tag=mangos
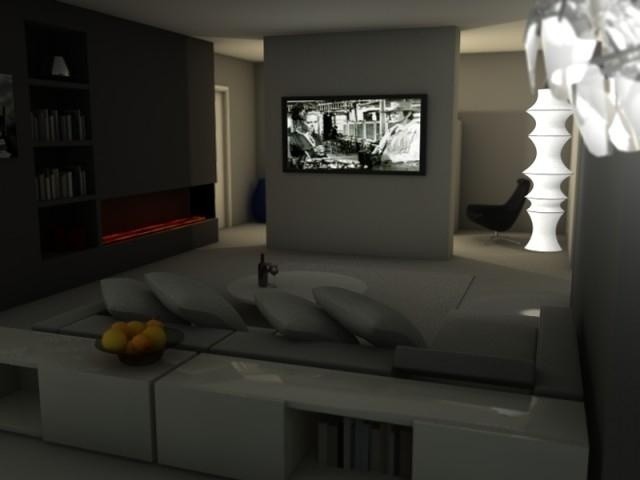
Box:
[102,319,166,353]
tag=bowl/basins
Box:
[95,325,186,366]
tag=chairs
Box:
[465,178,530,246]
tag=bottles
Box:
[257,253,268,287]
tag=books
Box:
[29,105,97,203]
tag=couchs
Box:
[31,296,582,400]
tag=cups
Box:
[303,100,386,169]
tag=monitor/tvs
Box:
[280,93,429,177]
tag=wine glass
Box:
[269,264,279,288]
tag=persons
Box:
[370,99,420,164]
[288,109,328,170]
